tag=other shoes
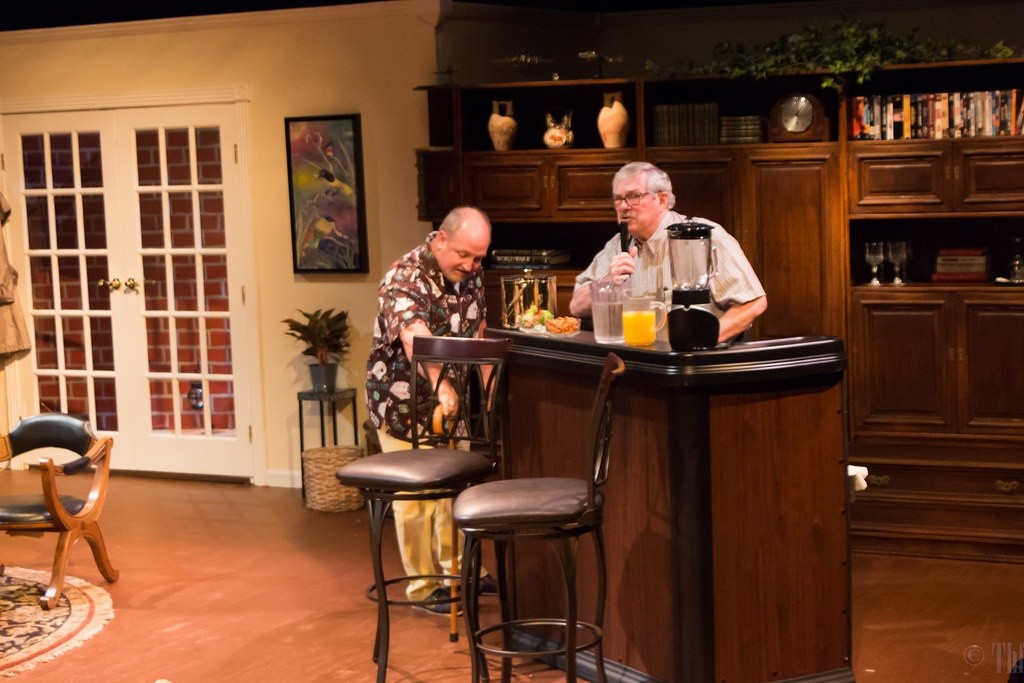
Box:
[411,587,465,616]
[443,575,500,595]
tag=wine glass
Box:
[866,243,883,287]
[888,242,906,287]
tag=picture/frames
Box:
[283,112,370,274]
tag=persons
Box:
[366,206,497,618]
[569,162,768,343]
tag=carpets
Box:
[0,565,116,679]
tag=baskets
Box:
[302,446,368,512]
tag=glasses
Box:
[608,190,664,208]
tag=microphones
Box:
[620,221,629,254]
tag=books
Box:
[647,103,762,146]
[851,89,1024,139]
[491,250,569,269]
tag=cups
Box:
[590,280,624,345]
[623,296,668,346]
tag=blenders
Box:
[665,222,728,352]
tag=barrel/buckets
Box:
[500,269,557,329]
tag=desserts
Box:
[544,315,580,335]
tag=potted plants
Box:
[281,307,353,394]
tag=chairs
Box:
[334,335,512,683]
[452,351,628,683]
[0,412,120,611]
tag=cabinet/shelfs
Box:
[296,387,358,499]
[415,56,1024,566]
